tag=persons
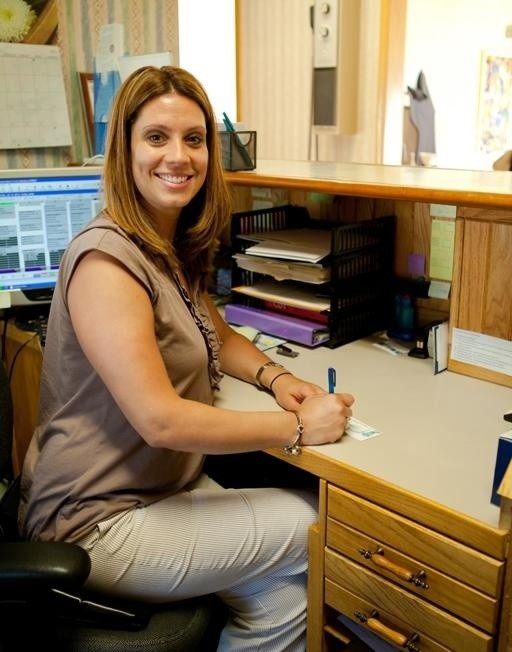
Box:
[14,65,358,651]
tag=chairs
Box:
[0,362,221,651]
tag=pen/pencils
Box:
[328,368,336,394]
[223,112,253,167]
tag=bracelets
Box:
[267,372,293,392]
[253,361,285,392]
[280,406,305,457]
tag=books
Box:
[222,223,379,348]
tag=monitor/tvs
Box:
[0,166,107,301]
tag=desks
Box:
[0,151,511,651]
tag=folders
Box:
[225,304,330,347]
